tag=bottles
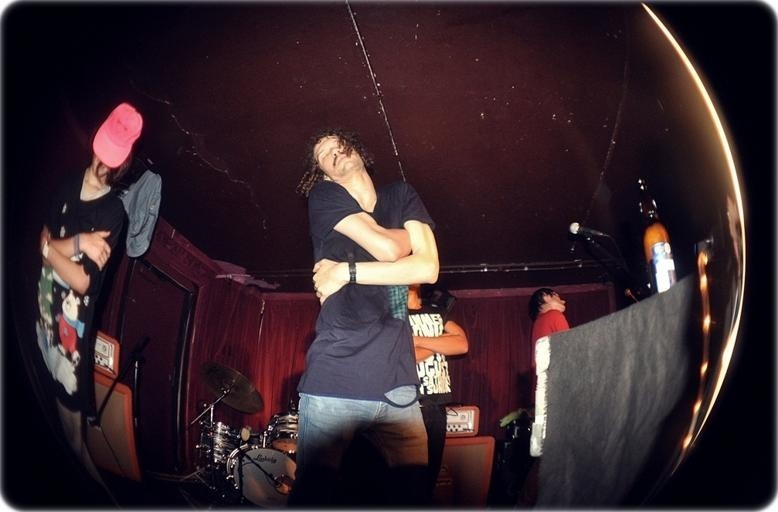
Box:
[651,242,677,295]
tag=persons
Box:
[521,285,576,465]
[40,99,146,470]
[282,129,448,493]
[400,279,470,465]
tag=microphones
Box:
[118,335,150,381]
[570,220,609,239]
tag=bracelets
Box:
[347,262,358,285]
[42,240,50,259]
[72,233,81,256]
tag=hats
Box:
[92,102,144,170]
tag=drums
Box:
[269,410,297,454]
[226,443,295,509]
[199,418,263,477]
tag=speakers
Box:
[434,436,496,508]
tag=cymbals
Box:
[200,361,263,414]
